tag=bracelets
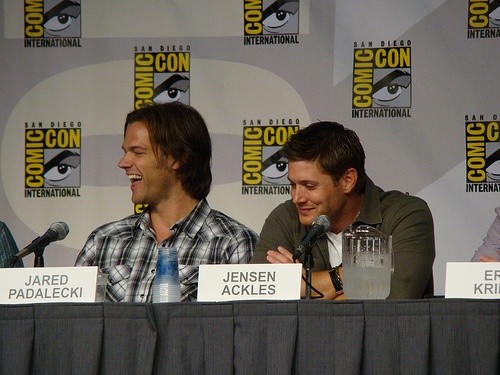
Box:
[329,267,344,296]
[335,267,343,289]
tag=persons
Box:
[249,121,436,300]
[470,207,500,262]
[72,100,260,303]
[0,220,27,268]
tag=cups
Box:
[152,247,181,303]
[96,274,106,304]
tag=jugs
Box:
[342,225,395,300]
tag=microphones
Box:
[292,215,331,260]
[10,222,70,266]
